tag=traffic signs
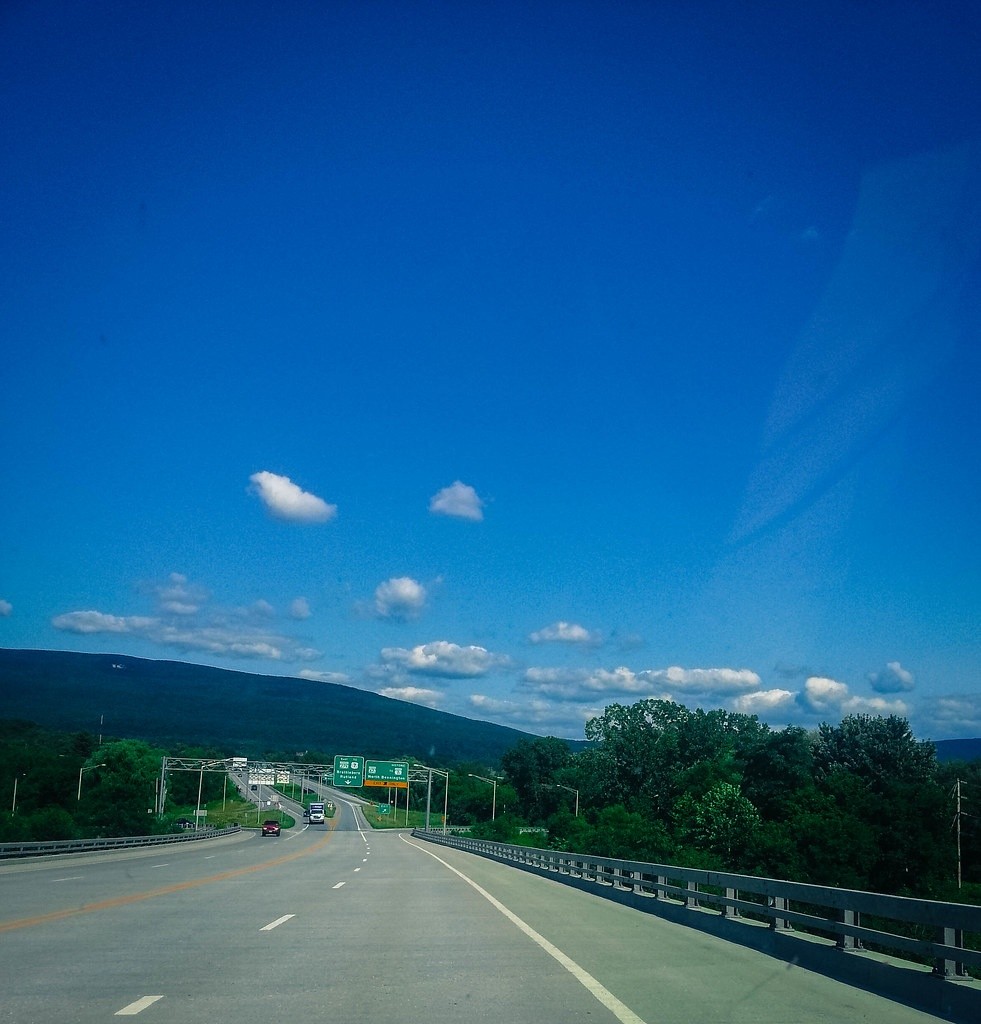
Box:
[364,759,409,788]
[333,756,364,787]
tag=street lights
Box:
[557,784,578,816]
[155,774,174,812]
[468,774,496,821]
[12,772,26,811]
[195,758,230,831]
[78,764,106,801]
[414,764,449,835]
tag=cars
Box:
[238,774,242,777]
[251,785,258,791]
[175,818,195,828]
[261,821,282,837]
[304,811,310,817]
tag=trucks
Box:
[308,802,325,824]
